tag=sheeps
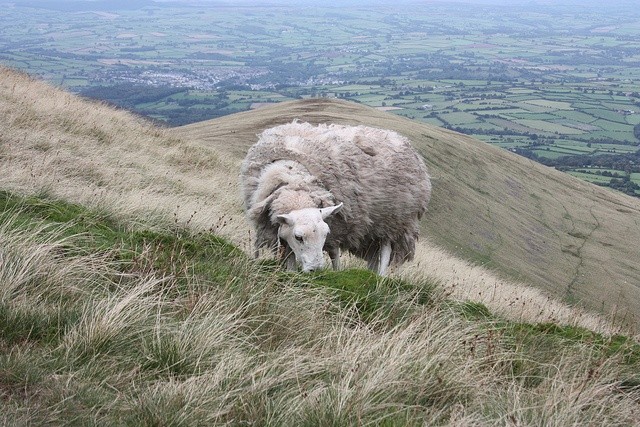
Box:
[240,119,431,280]
